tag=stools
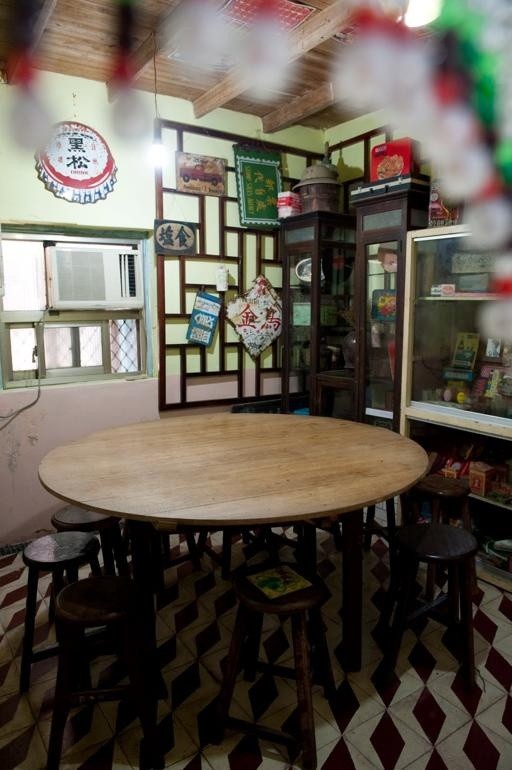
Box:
[410,475,481,612]
[377,521,480,705]
[44,576,165,770]
[203,556,338,770]
[120,516,366,580]
[43,502,132,629]
[16,529,103,710]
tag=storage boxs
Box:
[292,300,338,328]
[449,252,501,274]
[349,172,431,206]
[456,273,492,293]
[369,136,423,181]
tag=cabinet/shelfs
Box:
[277,190,429,434]
[400,226,512,594]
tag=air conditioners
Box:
[42,241,146,312]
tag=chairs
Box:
[229,398,283,414]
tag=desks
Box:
[34,407,433,742]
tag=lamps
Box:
[139,29,174,171]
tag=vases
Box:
[341,330,377,371]
